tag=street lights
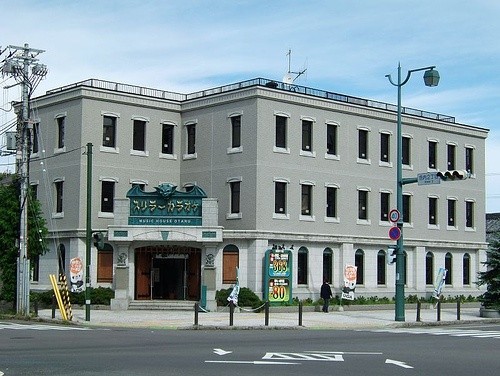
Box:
[384,61,440,321]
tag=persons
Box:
[320,279,333,313]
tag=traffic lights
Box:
[436,169,469,181]
